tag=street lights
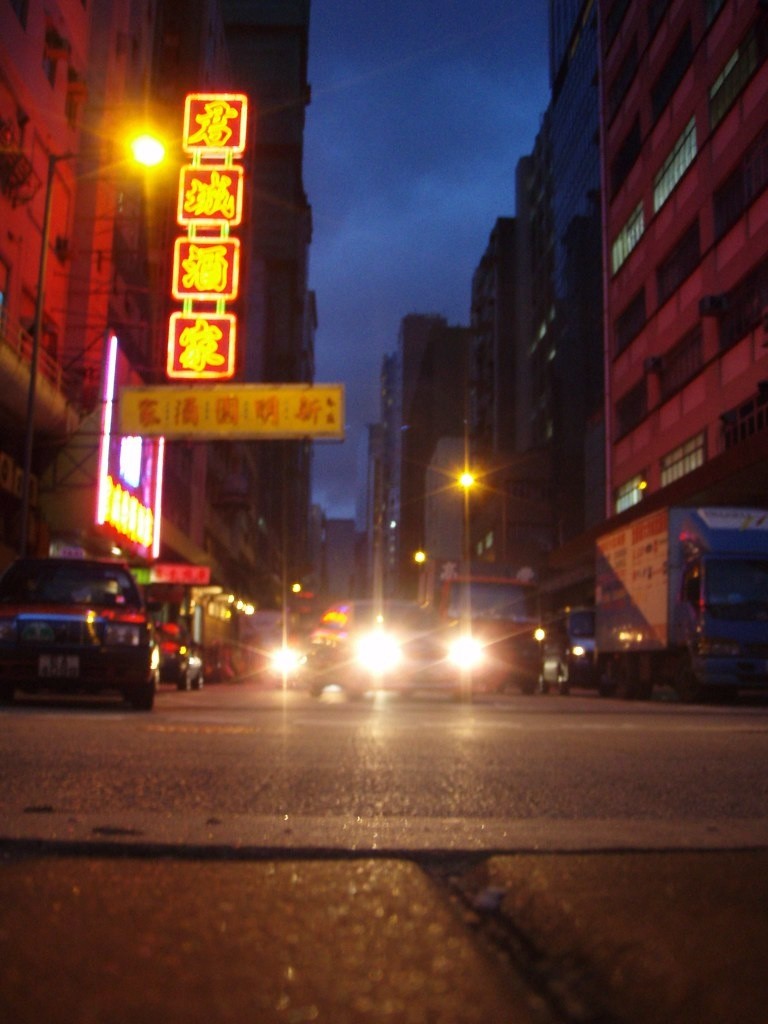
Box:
[19,133,168,560]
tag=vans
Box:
[538,606,595,695]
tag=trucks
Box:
[596,504,768,700]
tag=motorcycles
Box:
[441,577,543,696]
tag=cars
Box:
[0,557,158,709]
[310,596,474,705]
[153,629,205,690]
